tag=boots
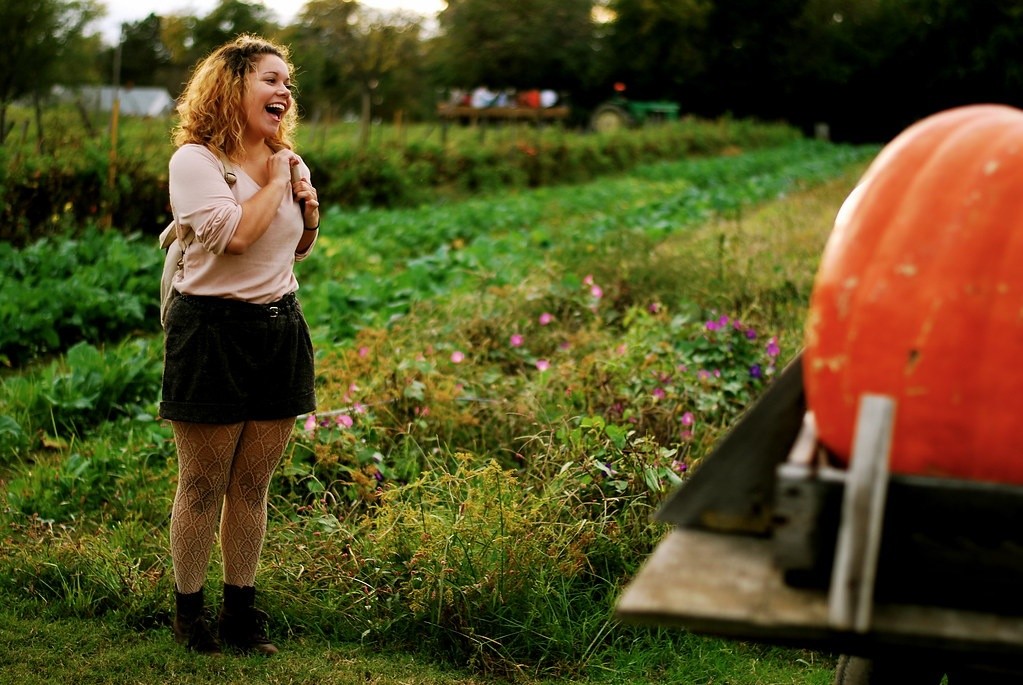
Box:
[173,581,221,655]
[217,584,271,648]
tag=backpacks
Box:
[159,145,302,330]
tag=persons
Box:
[159,36,320,655]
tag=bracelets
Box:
[304,222,319,231]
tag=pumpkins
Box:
[806,103,1022,488]
